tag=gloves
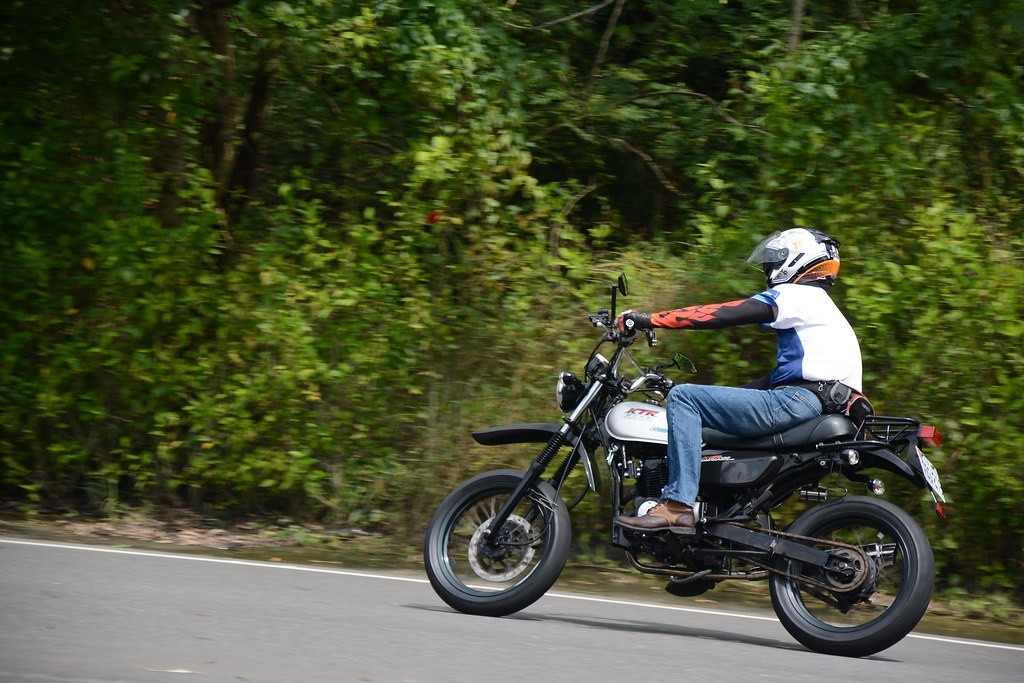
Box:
[616,309,653,336]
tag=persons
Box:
[613,227,864,598]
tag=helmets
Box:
[746,227,841,288]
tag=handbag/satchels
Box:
[793,379,876,424]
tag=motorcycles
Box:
[423,272,949,657]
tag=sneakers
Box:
[664,572,730,597]
[615,499,696,535]
